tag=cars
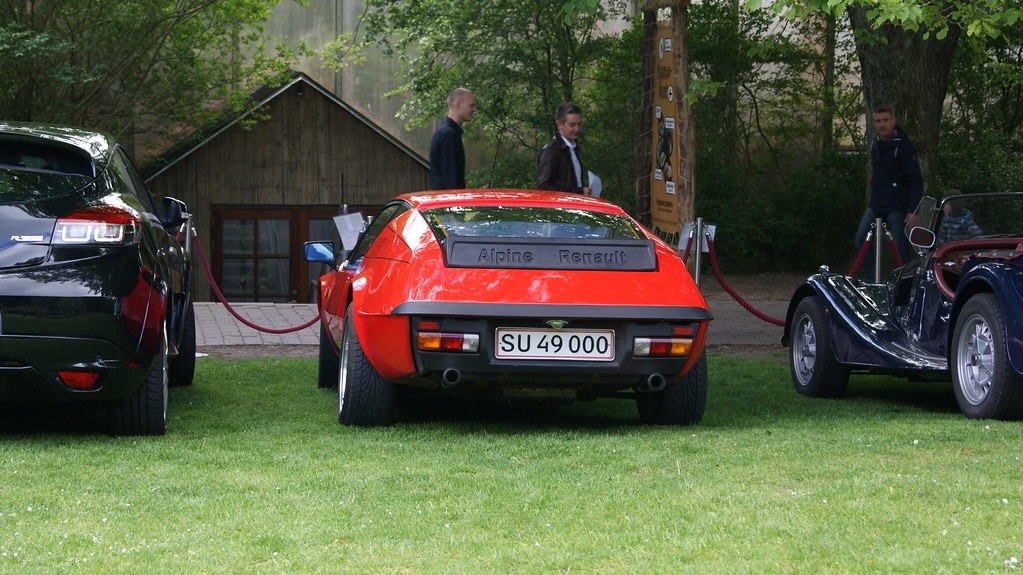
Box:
[1,122,198,434]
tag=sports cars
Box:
[780,190,1023,422]
[300,187,715,427]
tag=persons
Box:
[536,103,592,196]
[937,189,982,249]
[430,88,476,191]
[854,103,922,267]
